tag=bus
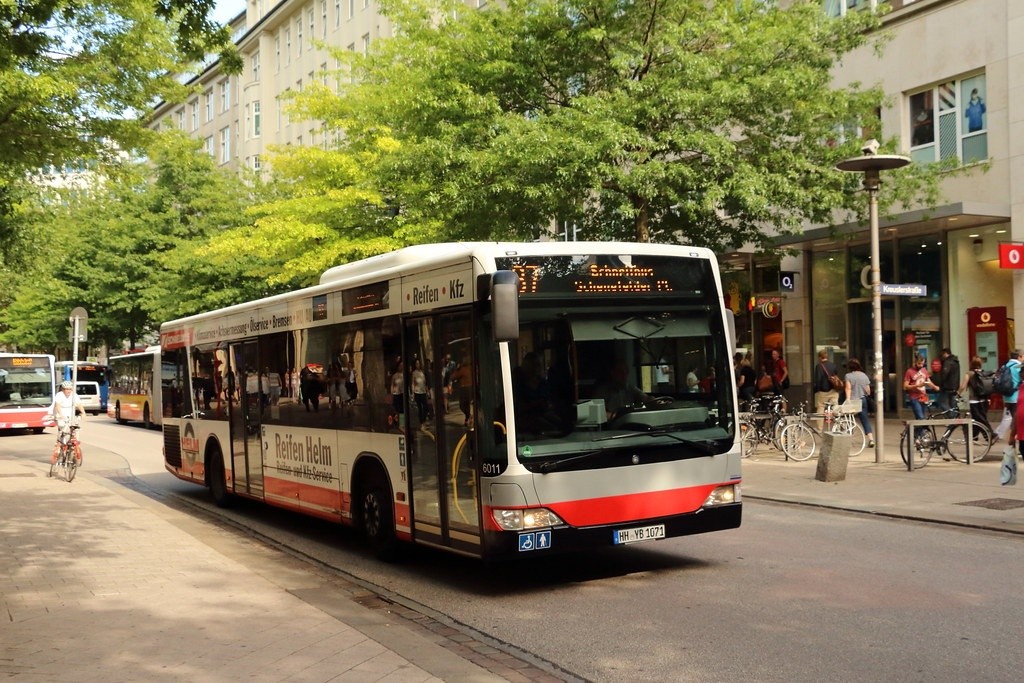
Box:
[103,344,164,430]
[0,353,56,434]
[158,238,745,559]
[54,360,109,411]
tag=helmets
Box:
[63,381,73,389]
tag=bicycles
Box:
[52,415,83,482]
[739,393,867,463]
[898,389,993,470]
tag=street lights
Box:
[835,139,913,464]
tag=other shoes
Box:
[990,434,998,444]
[868,441,874,448]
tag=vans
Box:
[56,380,102,416]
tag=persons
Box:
[815,350,840,433]
[934,348,960,430]
[366,352,476,435]
[514,352,570,428]
[49,381,86,478]
[844,358,875,447]
[900,354,940,439]
[995,348,1024,461]
[170,378,185,417]
[965,88,986,133]
[956,357,998,445]
[734,349,789,414]
[655,351,717,393]
[190,360,358,436]
[603,359,655,420]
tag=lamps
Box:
[920,239,927,247]
[973,238,983,254]
[936,238,943,246]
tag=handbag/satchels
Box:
[999,446,1017,485]
[781,375,790,390]
[829,376,843,388]
[757,374,773,390]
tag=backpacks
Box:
[968,368,993,396]
[991,363,1022,397]
